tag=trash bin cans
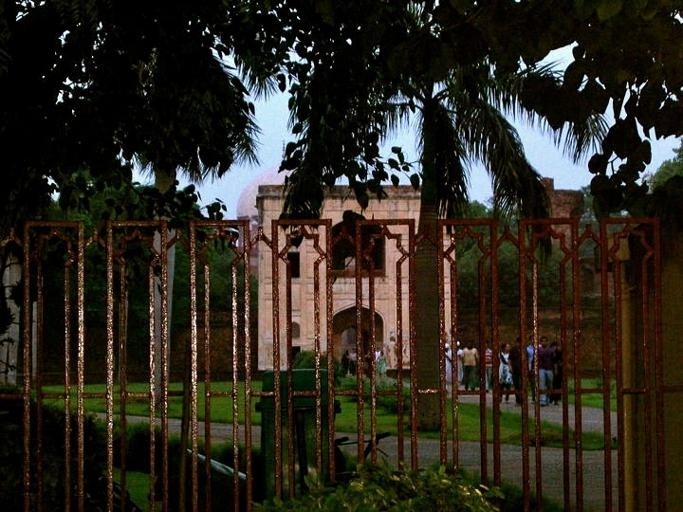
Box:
[256,368,341,501]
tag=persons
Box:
[445,334,563,407]
[342,336,398,377]
